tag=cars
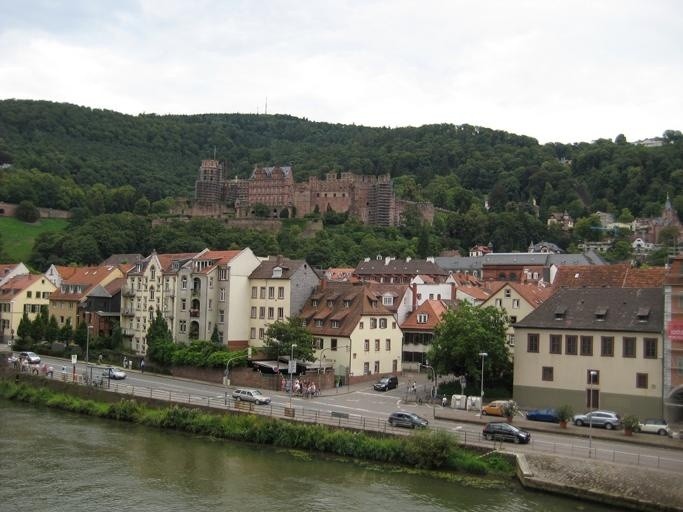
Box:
[104,368,125,379]
[19,351,40,364]
[482,400,510,416]
[526,408,560,423]
[632,418,668,435]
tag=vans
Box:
[374,375,398,392]
[232,388,271,405]
[387,412,428,429]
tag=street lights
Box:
[589,371,598,458]
[85,325,94,382]
[290,343,298,408]
[478,352,488,399]
[422,363,436,403]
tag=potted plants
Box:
[555,403,577,429]
[618,415,642,436]
[503,406,513,422]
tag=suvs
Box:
[572,409,620,429]
[483,422,530,443]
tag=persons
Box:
[122,355,128,369]
[98,352,104,365]
[280,377,321,400]
[140,357,145,374]
[9,357,66,378]
[440,391,447,409]
[414,380,417,388]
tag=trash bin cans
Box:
[223,377,230,385]
[71,355,77,364]
